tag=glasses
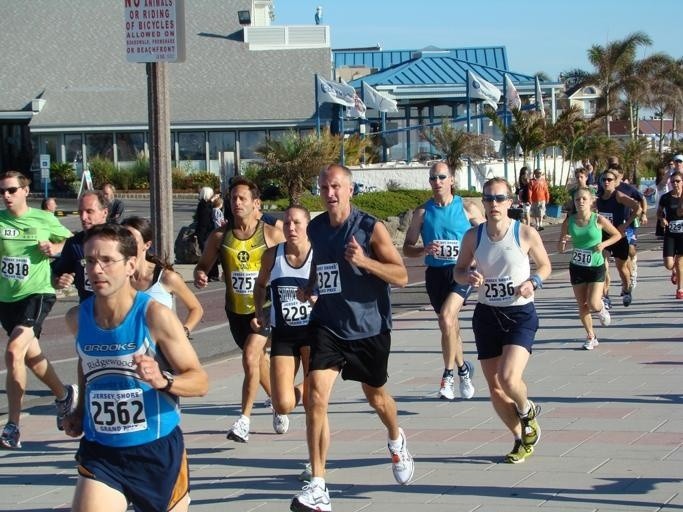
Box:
[429,175,452,181]
[602,178,613,182]
[80,256,130,268]
[671,180,682,183]
[0,185,26,195]
[483,195,510,203]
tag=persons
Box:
[516,153,683,351]
[404,163,488,402]
[64,222,209,510]
[194,181,291,443]
[291,163,416,511]
[195,186,284,281]
[452,177,553,464]
[1,168,79,451]
[41,177,205,341]
[252,203,314,480]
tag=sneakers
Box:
[0,422,22,449]
[298,462,313,481]
[270,398,289,434]
[461,362,475,400]
[388,427,414,487]
[518,400,540,447]
[504,441,533,463]
[291,483,332,512]
[54,384,79,430]
[583,255,683,351]
[228,420,248,444]
[438,376,455,401]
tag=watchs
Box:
[158,370,174,392]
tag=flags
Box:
[337,76,367,119]
[468,70,502,109]
[361,80,399,112]
[315,76,354,106]
[536,75,547,125]
[505,73,523,117]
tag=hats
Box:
[608,156,619,165]
[673,155,683,162]
[198,186,214,203]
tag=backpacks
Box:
[174,227,201,264]
